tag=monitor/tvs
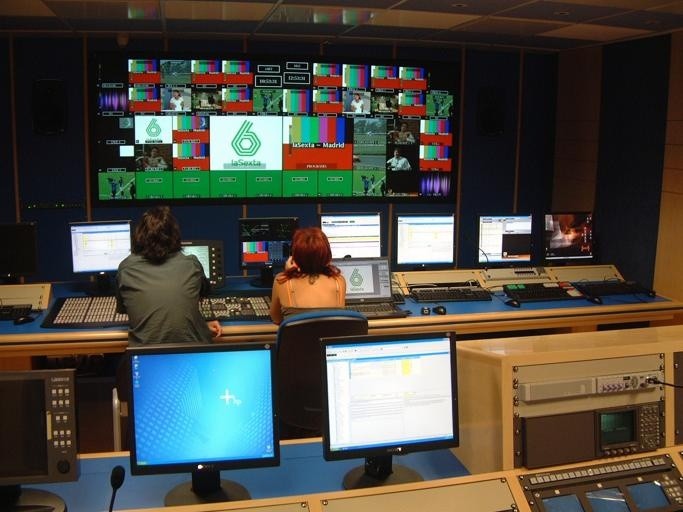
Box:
[393,211,455,271]
[473,211,536,270]
[91,49,460,209]
[0,368,78,512]
[180,239,226,289]
[318,328,461,490]
[319,212,384,258]
[66,220,134,294]
[0,221,37,276]
[237,216,300,287]
[540,210,596,265]
[124,342,282,507]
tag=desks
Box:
[0,264,683,370]
[1,429,682,512]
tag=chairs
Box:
[270,309,369,442]
[111,388,130,452]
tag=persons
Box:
[143,147,168,171]
[263,91,283,112]
[199,95,216,107]
[110,177,135,200]
[270,227,346,321]
[351,93,365,113]
[432,95,452,116]
[170,90,185,110]
[388,122,416,145]
[387,148,411,171]
[114,206,223,344]
[362,174,386,196]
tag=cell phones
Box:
[422,306,431,315]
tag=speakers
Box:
[31,78,69,134]
[475,86,503,140]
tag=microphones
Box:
[108,462,127,512]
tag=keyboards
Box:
[503,280,586,301]
[570,281,645,297]
[412,289,493,302]
[0,304,32,320]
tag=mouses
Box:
[591,296,602,304]
[504,299,520,308]
[649,290,655,298]
[14,315,35,325]
[433,305,446,314]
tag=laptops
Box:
[327,257,405,319]
